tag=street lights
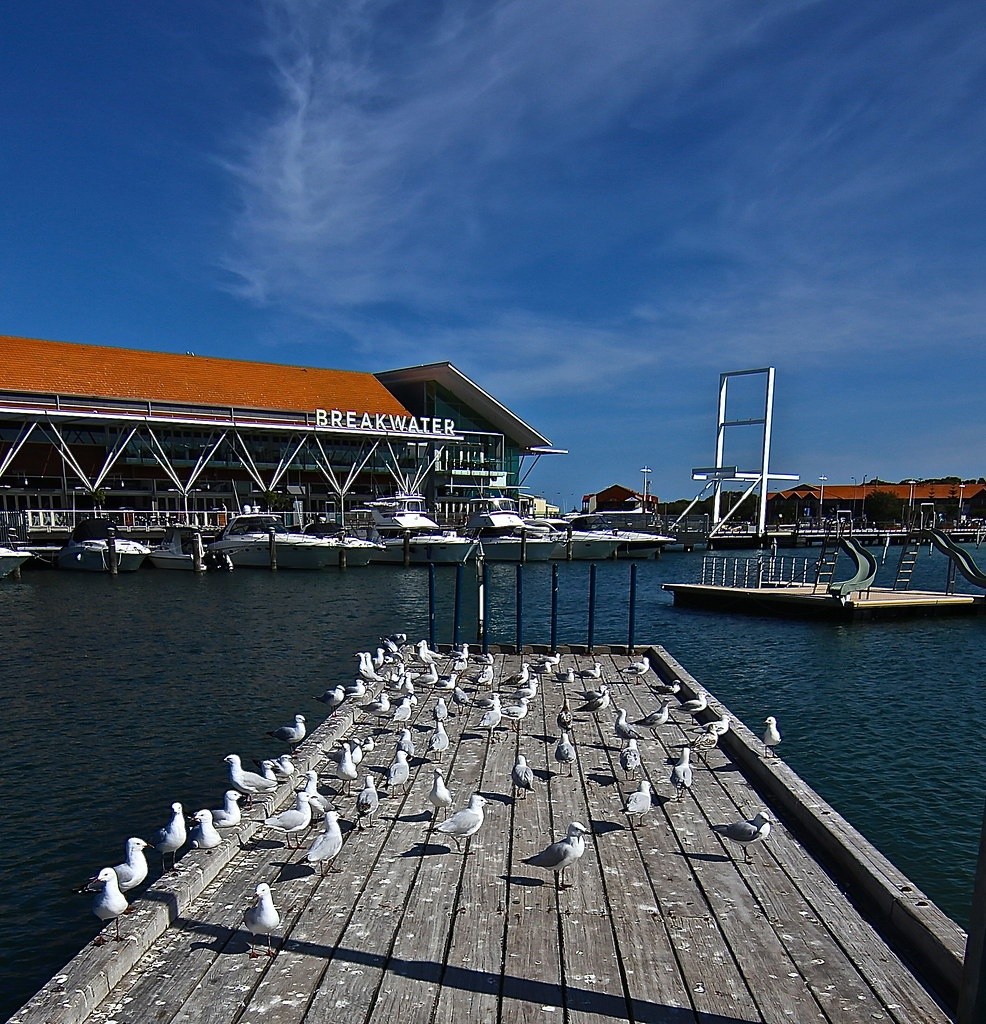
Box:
[640,466,653,515]
[906,480,916,530]
[861,474,867,521]
[957,484,965,528]
[850,476,856,519]
[819,474,827,530]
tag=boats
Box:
[523,518,623,560]
[465,498,559,561]
[345,495,480,564]
[149,525,196,571]
[563,515,677,558]
[56,517,152,572]
[206,514,380,569]
[0,546,33,576]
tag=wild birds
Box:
[86,637,787,965]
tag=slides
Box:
[827,535,878,600]
[918,528,985,590]
